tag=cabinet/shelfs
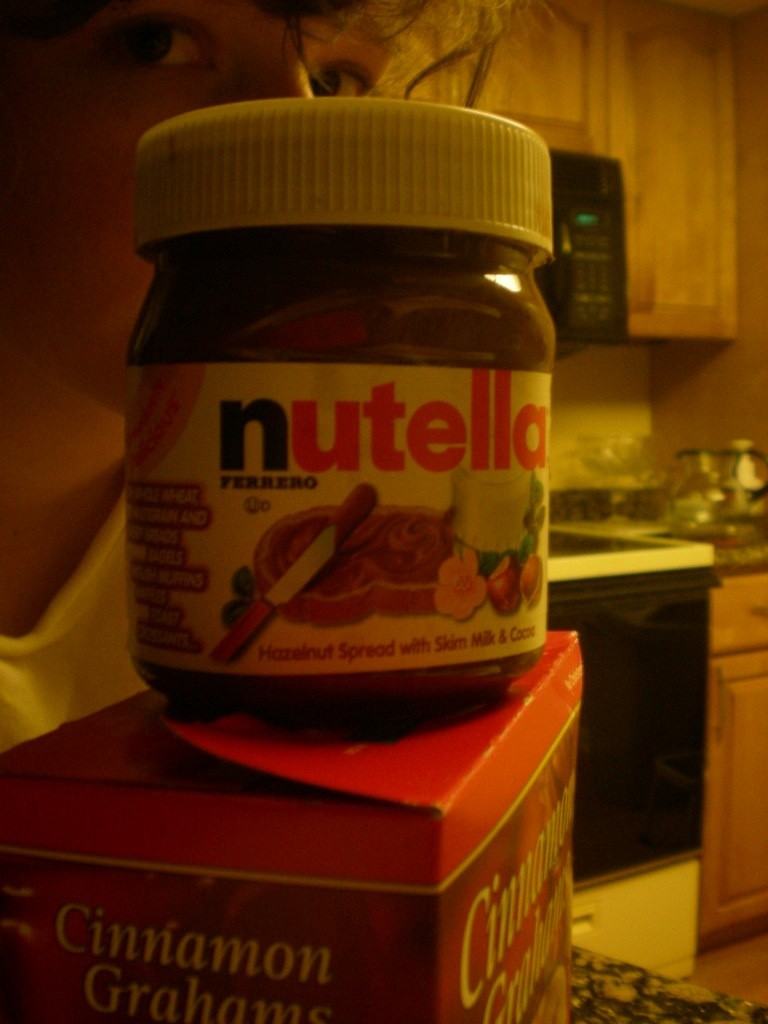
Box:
[290,0,739,345]
[700,568,768,949]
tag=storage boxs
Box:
[0,631,584,1024]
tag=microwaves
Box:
[533,150,624,344]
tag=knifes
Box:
[207,527,335,663]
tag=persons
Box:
[0,1,504,755]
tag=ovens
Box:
[547,568,722,892]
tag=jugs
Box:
[666,450,768,539]
